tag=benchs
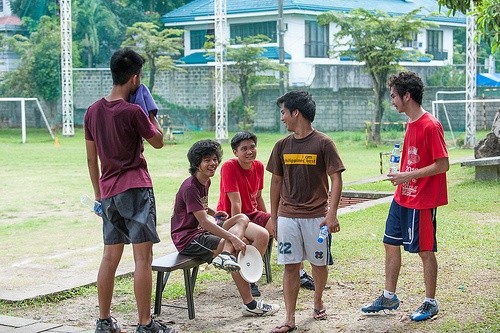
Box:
[461,156,500,181]
[152,233,273,320]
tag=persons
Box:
[216,131,331,297]
[359,71,449,322]
[170,139,281,317]
[84,47,175,333]
[266,91,346,333]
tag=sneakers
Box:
[250,282,260,296]
[94,315,121,333]
[410,301,439,322]
[297,271,315,289]
[212,253,241,273]
[136,319,175,333]
[242,301,279,317]
[361,291,401,313]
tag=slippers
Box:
[269,324,297,333]
[313,308,327,320]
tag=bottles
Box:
[217,217,224,227]
[389,144,400,179]
[318,225,329,243]
[81,195,104,216]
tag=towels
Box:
[128,83,158,117]
